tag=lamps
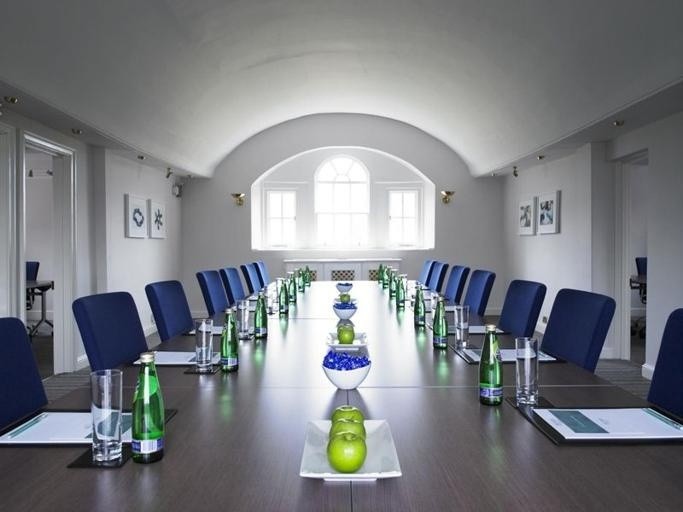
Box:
[232,194,244,205]
[441,191,454,203]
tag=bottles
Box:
[378,264,503,405]
[132,264,311,463]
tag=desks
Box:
[0,279,683,512]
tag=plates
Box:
[299,418,402,482]
[333,298,357,305]
[327,332,367,351]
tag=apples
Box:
[329,419,365,440]
[327,432,365,472]
[337,323,354,343]
[340,294,350,302]
[331,406,364,425]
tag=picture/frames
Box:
[148,198,168,240]
[536,188,560,234]
[125,192,147,238]
[517,196,536,236]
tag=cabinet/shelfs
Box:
[283,258,403,280]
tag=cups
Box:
[276,277,283,302]
[194,318,213,371]
[287,271,294,293]
[387,266,539,409]
[91,369,123,467]
[237,300,249,337]
[264,285,272,313]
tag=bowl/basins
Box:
[333,306,357,320]
[321,361,371,390]
[336,284,353,293]
